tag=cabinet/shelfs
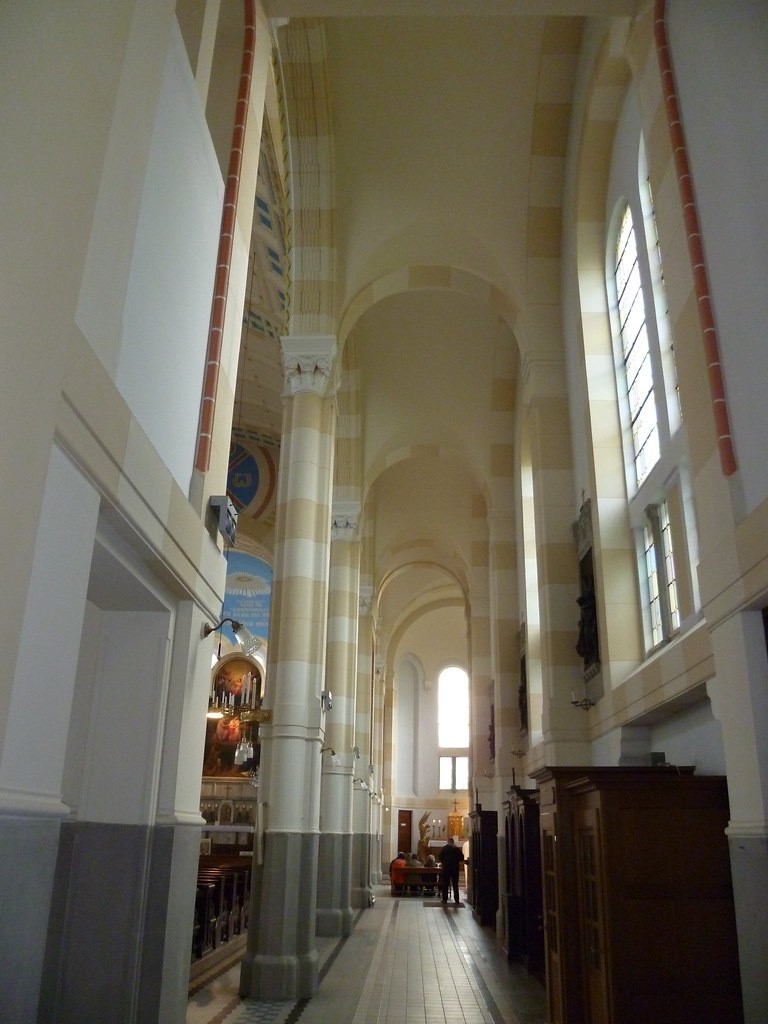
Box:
[560,773,744,1024]
[526,765,696,1024]
[502,768,545,965]
[468,788,499,927]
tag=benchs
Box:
[392,866,452,899]
[192,855,251,963]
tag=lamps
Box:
[233,722,254,766]
[203,619,262,657]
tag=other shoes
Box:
[455,901,460,904]
[441,900,447,903]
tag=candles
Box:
[240,671,256,709]
[212,690,218,709]
[438,820,441,827]
[222,691,234,707]
[432,819,435,825]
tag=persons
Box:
[389,837,464,905]
